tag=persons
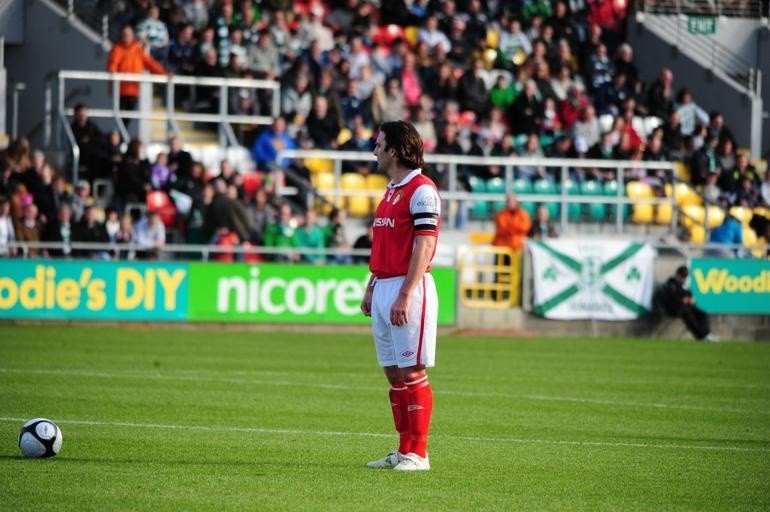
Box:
[653,264,722,344]
[1,1,769,261]
[358,118,444,474]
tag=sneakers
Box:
[366,450,405,467]
[393,452,431,471]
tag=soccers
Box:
[18,418,63,458]
